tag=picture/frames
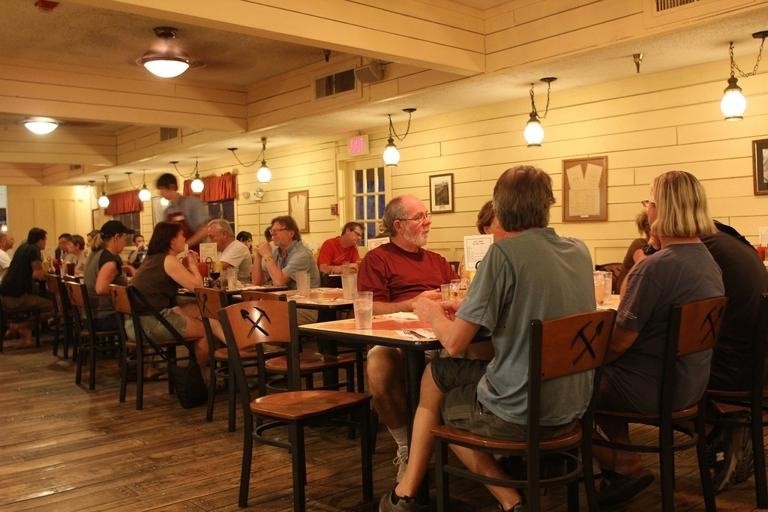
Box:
[562,156,607,221]
[752,139,768,195]
[288,190,309,233]
[429,173,454,214]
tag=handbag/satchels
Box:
[173,365,207,408]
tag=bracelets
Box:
[642,245,655,255]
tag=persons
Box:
[357,194,510,485]
[157,174,209,263]
[592,171,726,510]
[458,200,508,277]
[0,216,364,392]
[619,219,768,495]
[377,165,596,512]
[614,209,651,293]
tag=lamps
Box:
[18,116,60,135]
[382,115,401,166]
[523,84,545,147]
[97,181,110,208]
[256,137,272,183]
[135,26,192,78]
[720,42,747,121]
[138,169,151,202]
[160,197,169,207]
[191,161,205,193]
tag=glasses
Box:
[400,213,431,222]
[641,200,656,208]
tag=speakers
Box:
[354,63,383,84]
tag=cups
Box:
[351,290,373,329]
[593,271,612,307]
[440,279,468,303]
[227,268,239,291]
[296,271,311,295]
[341,273,357,301]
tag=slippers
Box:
[599,470,653,503]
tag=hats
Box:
[100,220,134,235]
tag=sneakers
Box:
[709,435,754,494]
[379,446,416,512]
[267,379,288,392]
